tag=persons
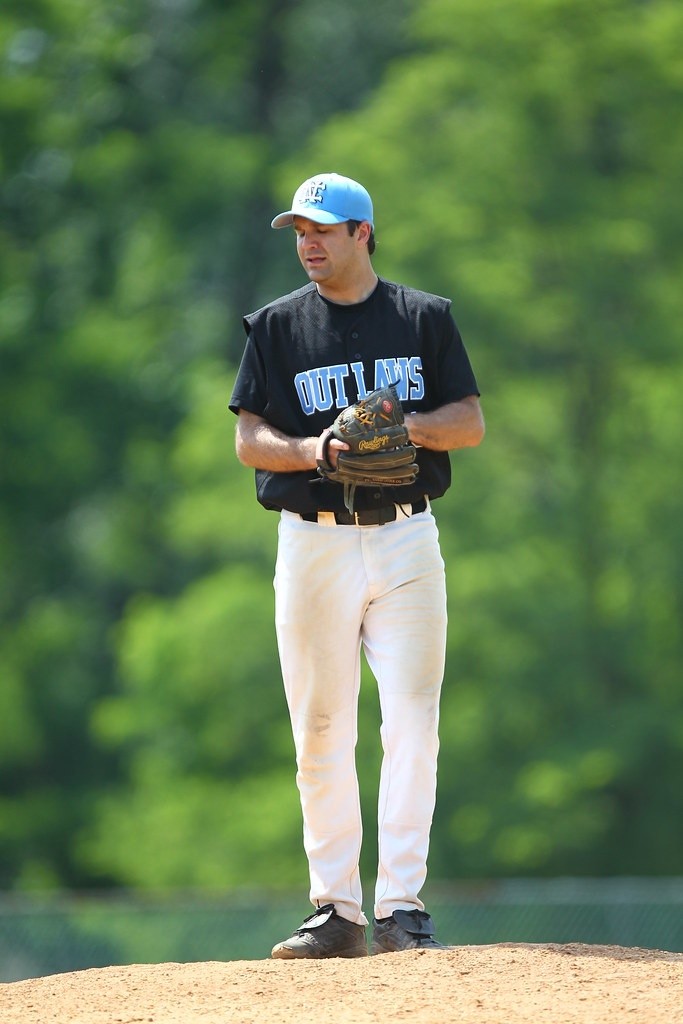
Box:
[229,176,484,959]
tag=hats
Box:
[272,173,374,229]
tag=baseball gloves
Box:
[315,382,418,491]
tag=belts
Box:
[301,498,427,526]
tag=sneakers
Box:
[272,904,367,960]
[371,909,442,954]
